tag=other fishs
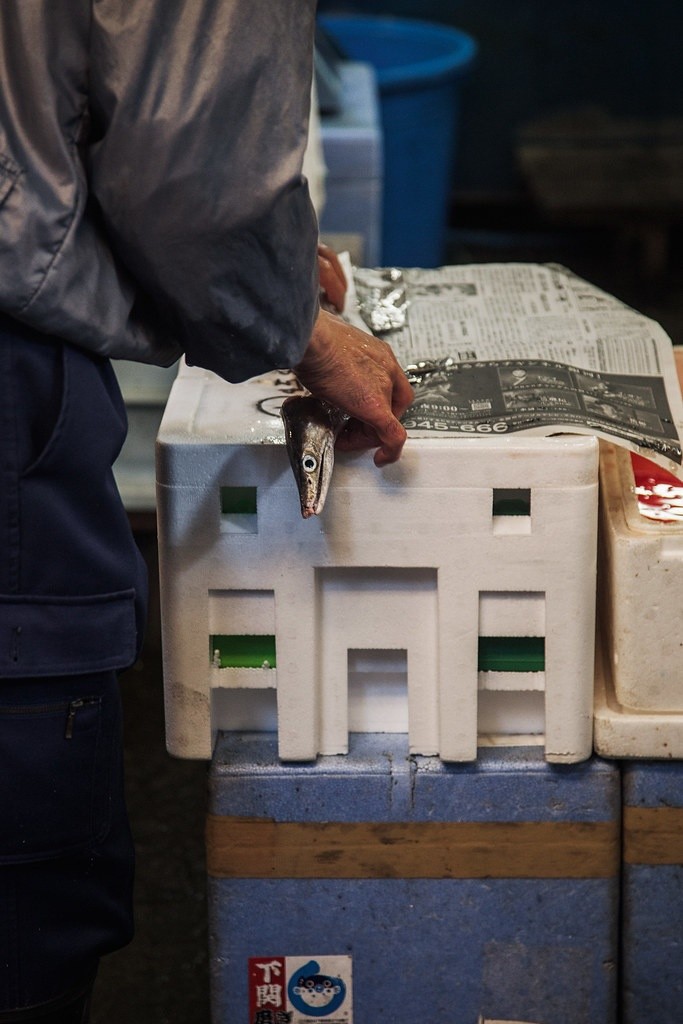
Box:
[280,293,349,519]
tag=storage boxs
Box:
[111,59,683,1024]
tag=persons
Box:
[0,0,411,1024]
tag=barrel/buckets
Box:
[314,13,479,272]
